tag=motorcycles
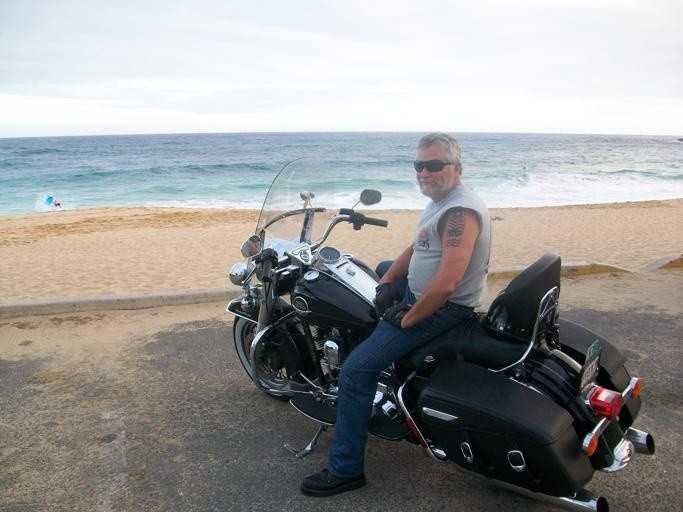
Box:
[226,157,655,512]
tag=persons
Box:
[298,131,494,500]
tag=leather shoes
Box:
[301,469,366,497]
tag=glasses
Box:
[412,160,455,172]
